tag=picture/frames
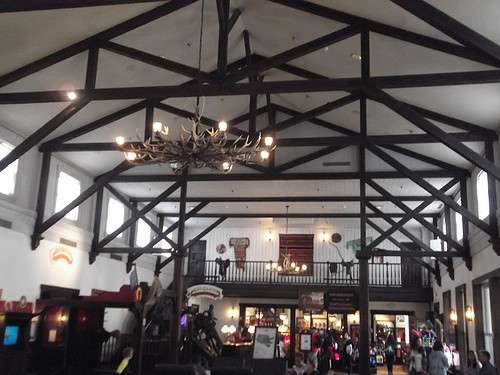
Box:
[299,333,312,352]
[251,326,278,359]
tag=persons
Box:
[477,350,496,375]
[238,321,455,375]
[464,350,480,375]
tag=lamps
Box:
[59,308,66,324]
[465,305,473,322]
[450,309,458,325]
[231,306,234,319]
[114,0,277,174]
[221,325,236,346]
[264,205,307,275]
[247,309,358,334]
[323,232,326,241]
[269,231,272,241]
